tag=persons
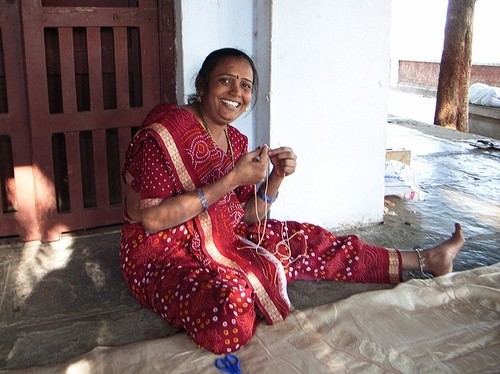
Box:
[118,47,466,356]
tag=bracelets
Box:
[196,188,209,211]
[257,183,279,203]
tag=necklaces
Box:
[197,102,235,170]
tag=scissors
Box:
[215,355,242,374]
